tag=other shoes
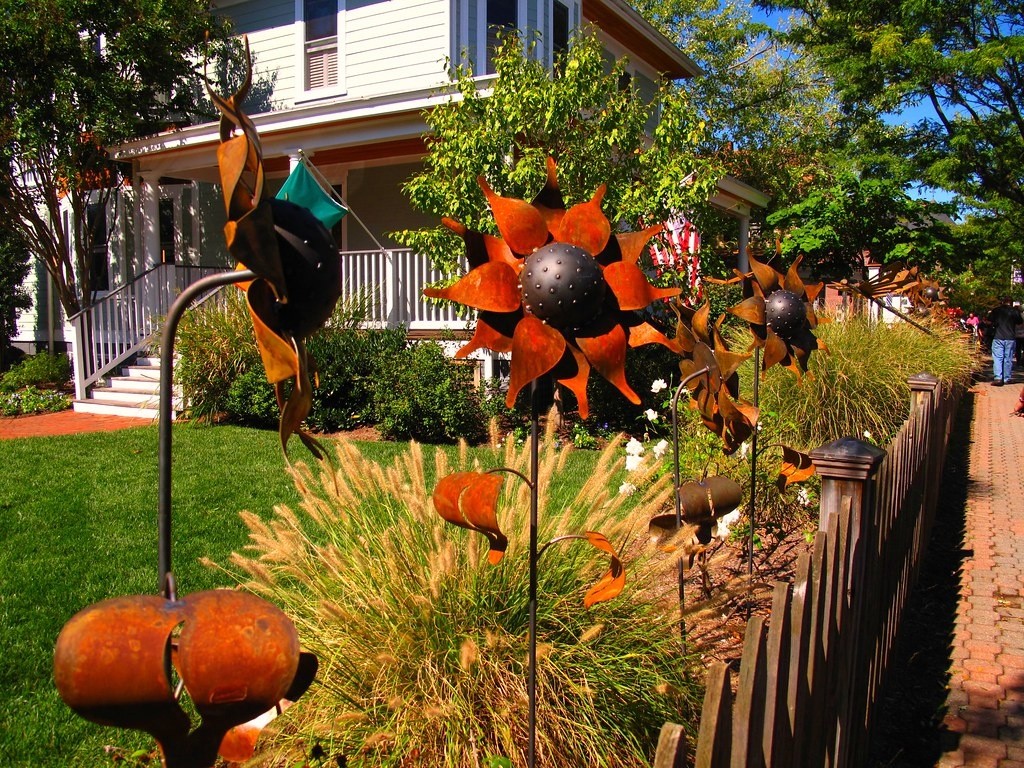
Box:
[990,380,1003,386]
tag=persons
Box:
[983,296,1024,386]
[1014,386,1024,418]
[967,313,979,337]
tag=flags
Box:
[275,160,350,232]
[638,173,702,314]
